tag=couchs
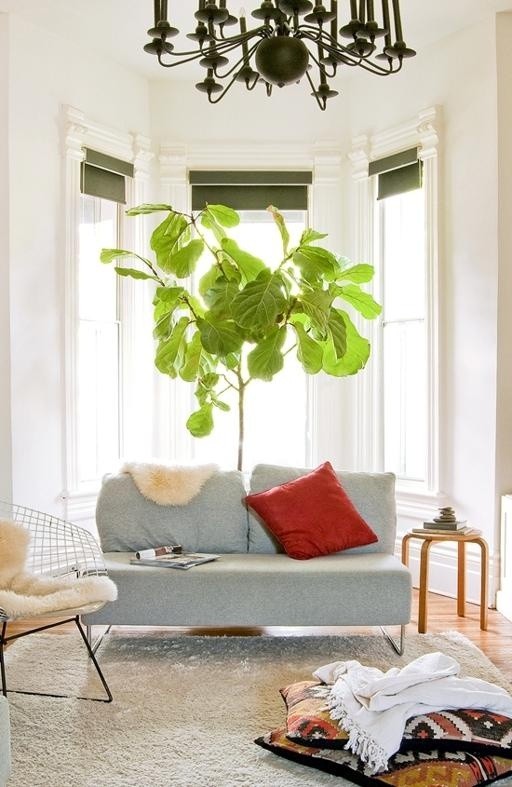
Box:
[72,461,413,656]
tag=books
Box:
[424,521,468,531]
[412,527,473,535]
[130,544,222,570]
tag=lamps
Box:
[142,1,416,113]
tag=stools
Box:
[399,527,490,636]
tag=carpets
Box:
[2,628,512,786]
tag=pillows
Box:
[239,460,379,560]
[252,670,512,787]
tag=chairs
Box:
[0,498,118,703]
